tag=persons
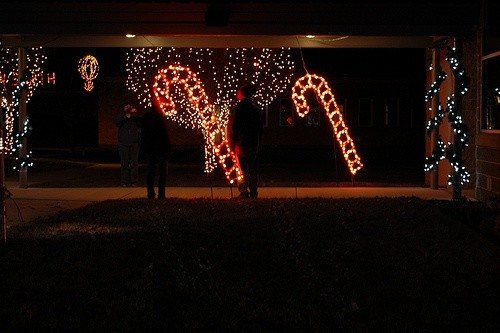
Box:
[227,86,263,198]
[140,106,169,200]
[113,100,140,185]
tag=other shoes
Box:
[250,192,258,199]
[158,193,165,200]
[148,193,155,199]
[240,191,248,198]
